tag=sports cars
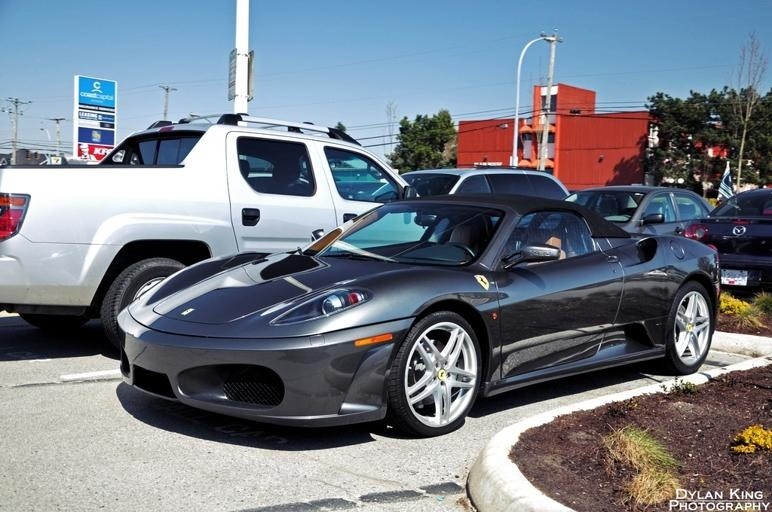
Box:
[112,192,722,436]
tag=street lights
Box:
[1,95,32,166]
[512,30,565,173]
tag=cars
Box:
[331,165,772,299]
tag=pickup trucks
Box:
[2,114,440,351]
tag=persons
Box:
[77,143,97,161]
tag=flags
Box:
[716,161,734,199]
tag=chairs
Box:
[240,159,309,196]
[450,224,566,264]
[598,199,665,215]
